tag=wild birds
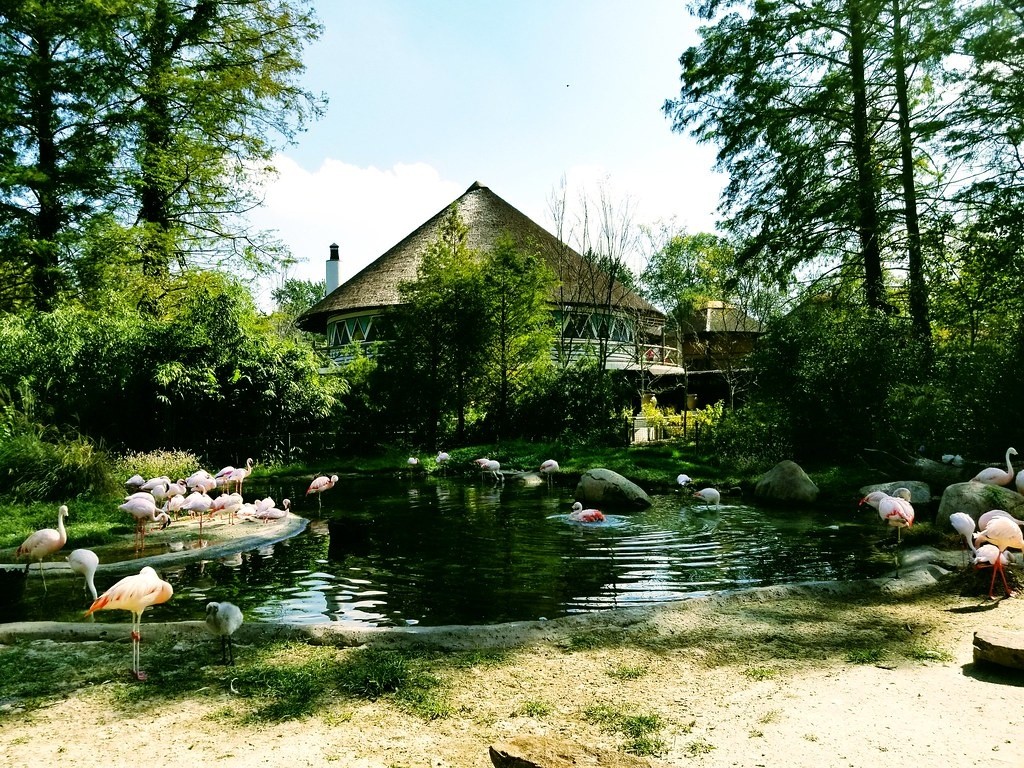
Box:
[15,457,292,680]
[305,474,339,502]
[407,457,420,472]
[473,458,505,483]
[435,450,451,472]
[941,452,963,468]
[540,458,559,480]
[948,509,1024,601]
[676,473,695,487]
[859,486,917,547]
[569,501,605,523]
[691,487,721,510]
[964,448,1019,486]
